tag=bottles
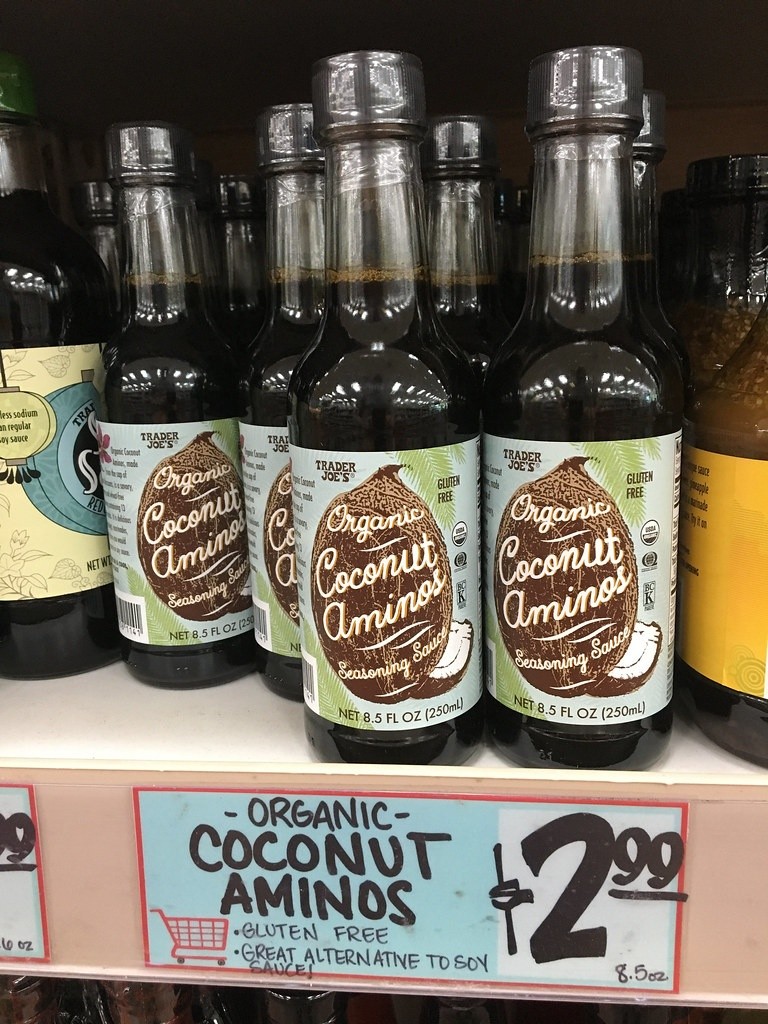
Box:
[480,41,686,770]
[288,48,487,766]
[1,49,676,744]
[676,152,767,766]
